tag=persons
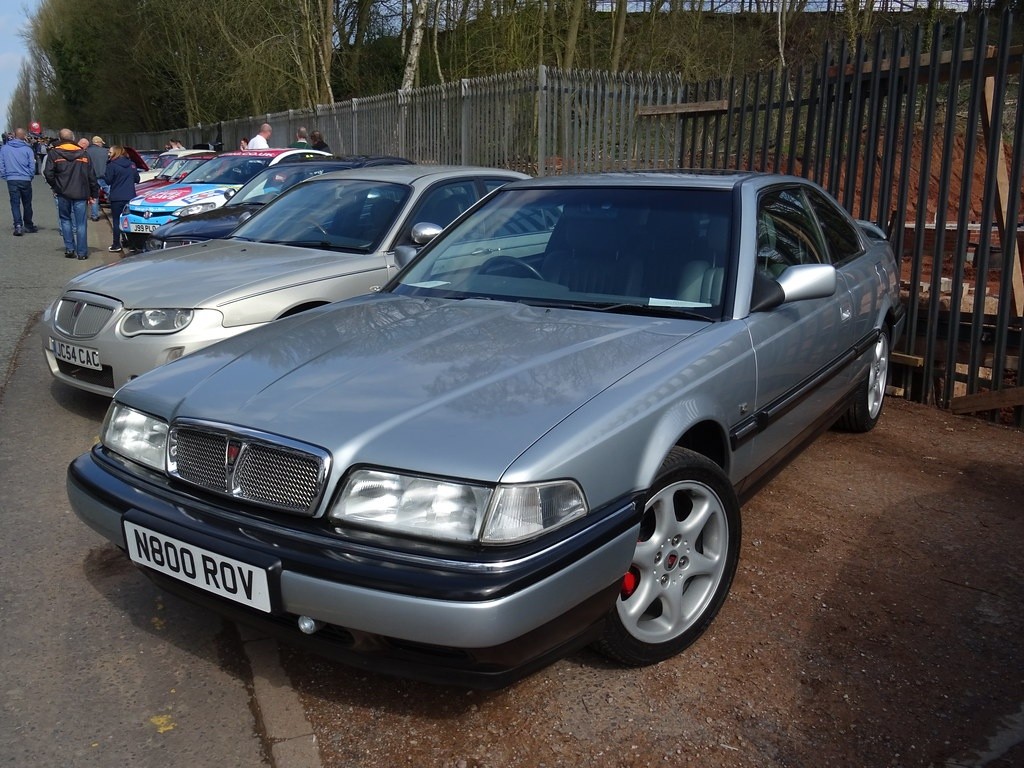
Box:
[239,123,332,154]
[165,138,187,153]
[0,126,141,261]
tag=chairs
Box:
[420,193,470,230]
[669,208,790,308]
[357,198,399,242]
[540,206,642,298]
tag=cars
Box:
[144,155,417,252]
[66,167,908,668]
[118,147,335,250]
[100,150,222,208]
[41,166,564,399]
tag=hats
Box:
[92,136,105,144]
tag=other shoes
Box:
[130,247,136,251]
[24,225,38,232]
[14,228,23,235]
[65,251,75,257]
[77,255,86,259]
[91,218,98,221]
[109,245,121,251]
